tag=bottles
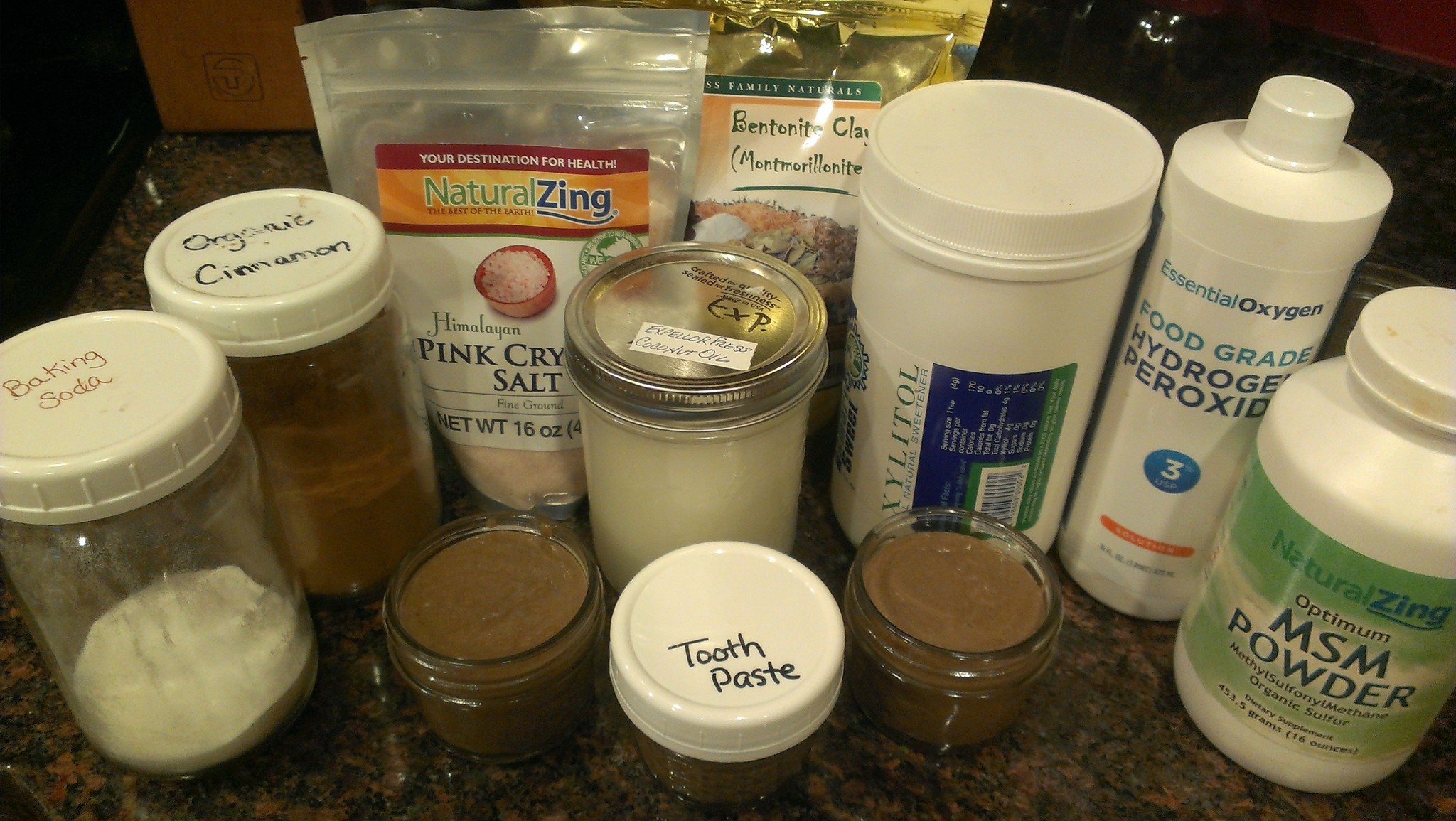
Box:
[841,506,1062,758]
[1055,72,1392,622]
[1172,287,1456,796]
[380,510,605,765]
